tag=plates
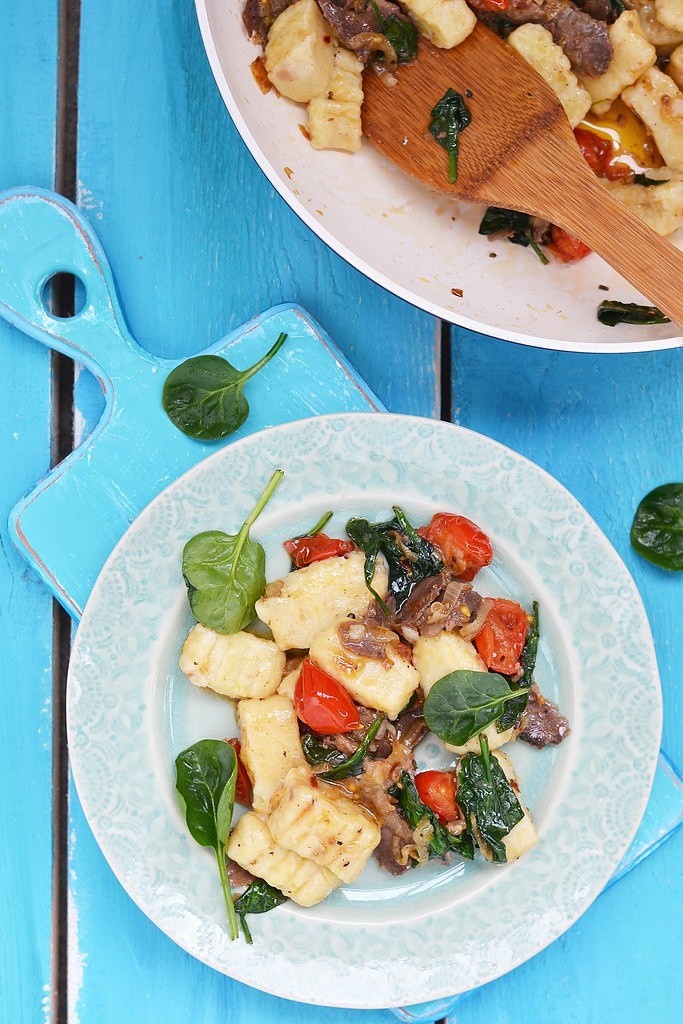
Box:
[193,1,681,354]
[66,412,664,1009]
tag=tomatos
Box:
[296,657,364,730]
[415,509,497,581]
[544,130,611,267]
[472,596,523,679]
[284,532,356,569]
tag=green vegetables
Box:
[316,716,385,785]
[184,467,283,635]
[160,329,292,444]
[375,2,546,263]
[383,602,544,864]
[630,480,683,572]
[175,737,283,942]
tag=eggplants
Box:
[223,733,255,811]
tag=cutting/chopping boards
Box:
[2,185,682,1024]
